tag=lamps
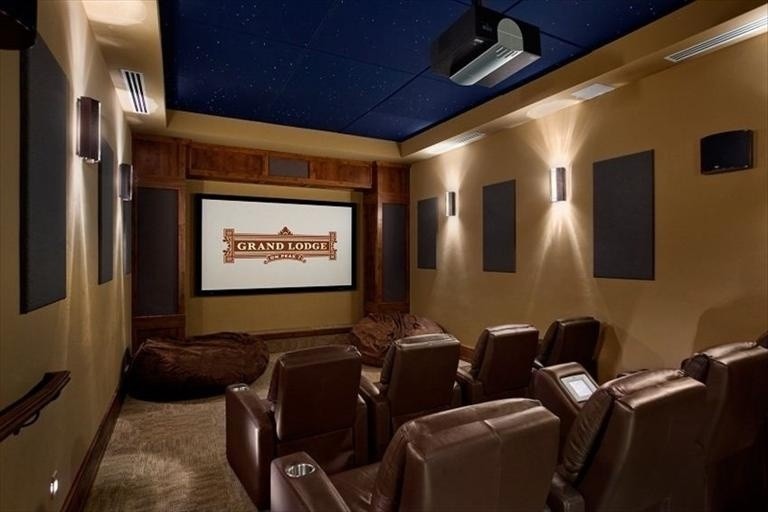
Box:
[549,167,567,202]
[76,96,103,164]
[447,191,455,216]
[118,163,134,201]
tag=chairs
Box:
[534,314,607,384]
[225,345,368,512]
[617,341,768,511]
[455,323,545,403]
[359,333,474,447]
[533,360,708,511]
[269,397,560,511]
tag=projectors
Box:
[430,5,543,91]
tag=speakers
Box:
[700,129,754,177]
